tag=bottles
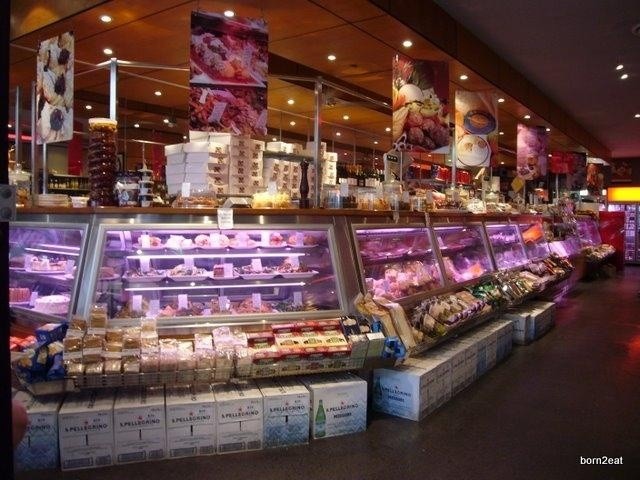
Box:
[321,180,411,210]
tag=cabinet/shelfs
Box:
[160,128,340,200]
[9,203,600,341]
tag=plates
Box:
[457,134,490,167]
[463,110,496,134]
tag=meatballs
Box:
[407,110,450,150]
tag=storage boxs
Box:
[375,311,533,427]
[14,370,368,471]
